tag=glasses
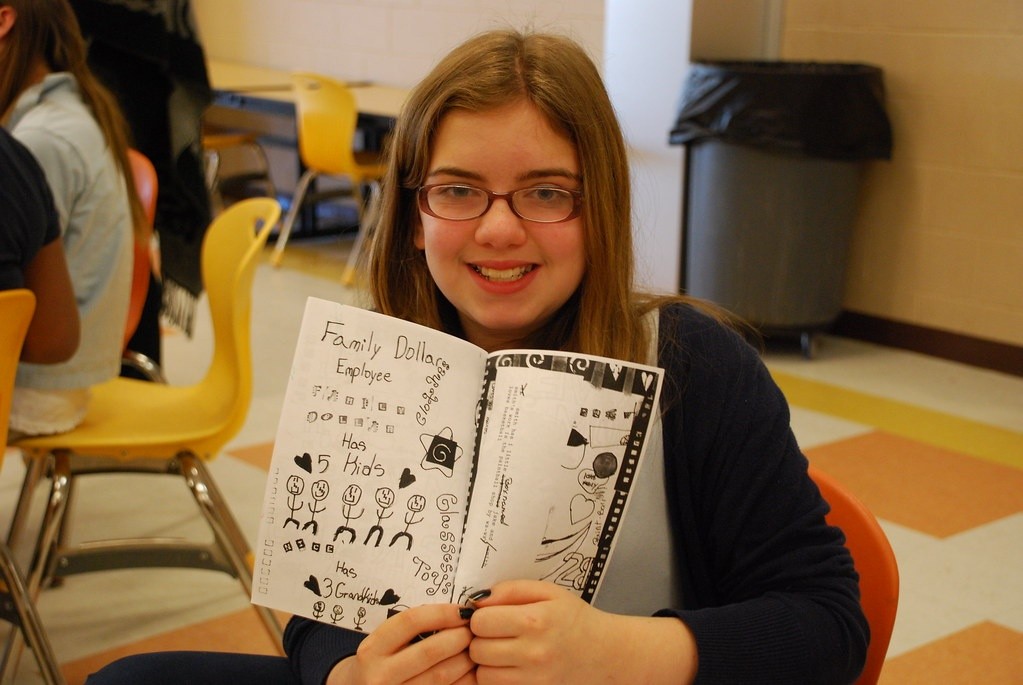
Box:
[416,180,584,223]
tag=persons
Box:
[0,122,81,366]
[86,27,873,685]
[0,0,148,444]
[71,0,219,385]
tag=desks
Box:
[235,85,416,241]
[206,64,370,244]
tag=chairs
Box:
[0,287,66,685]
[0,198,279,685]
[270,73,389,286]
[202,129,276,215]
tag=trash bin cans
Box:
[684,59,882,326]
